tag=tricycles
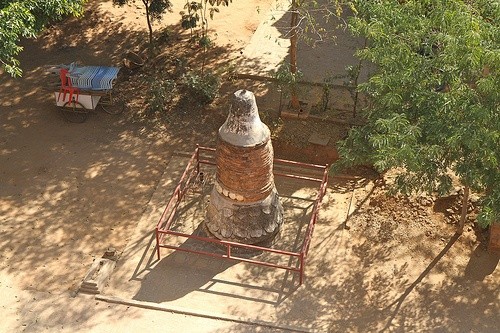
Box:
[54,78,125,123]
